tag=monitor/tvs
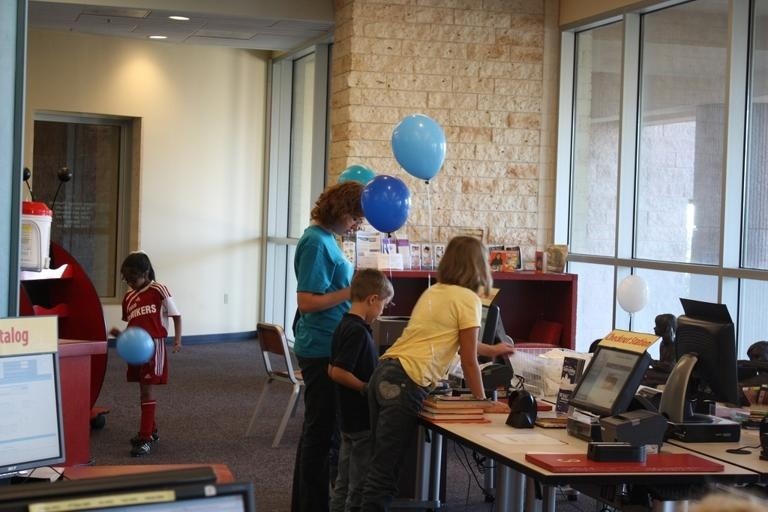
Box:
[477,303,514,401]
[658,315,741,444]
[568,350,651,442]
[0,353,64,481]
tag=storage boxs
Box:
[367,255,402,269]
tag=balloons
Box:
[362,175,412,238]
[391,114,446,185]
[617,275,649,317]
[116,327,156,364]
[339,165,375,186]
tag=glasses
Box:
[121,275,142,286]
[351,215,363,227]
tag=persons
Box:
[360,237,516,511]
[327,268,395,512]
[109,251,182,456]
[292,182,367,512]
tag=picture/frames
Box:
[487,245,504,272]
[435,245,445,267]
[409,244,420,268]
[422,244,433,271]
[507,246,522,271]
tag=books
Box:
[419,392,493,424]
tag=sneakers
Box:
[132,442,152,455]
[132,432,158,446]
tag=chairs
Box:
[245,323,306,448]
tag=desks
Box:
[419,398,759,512]
[522,394,768,487]
[61,464,237,512]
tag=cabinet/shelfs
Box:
[361,270,578,356]
[23,339,108,467]
